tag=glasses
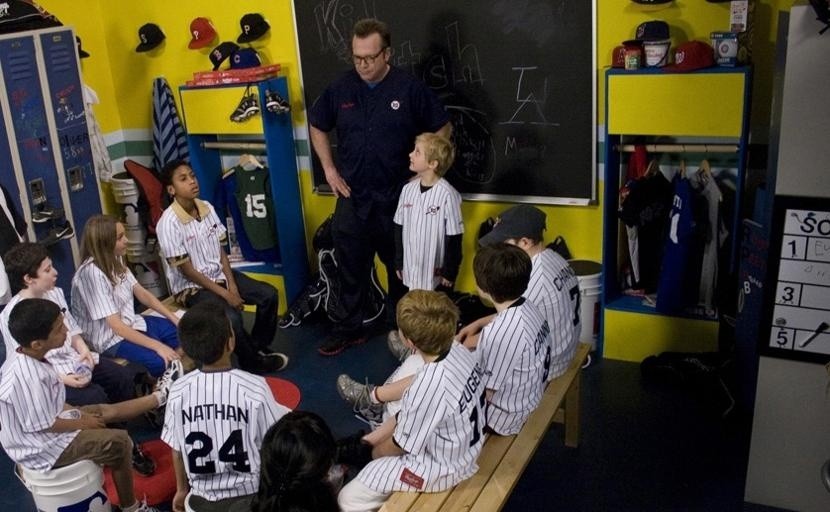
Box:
[352,48,387,64]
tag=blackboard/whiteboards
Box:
[292,0,598,206]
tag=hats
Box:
[210,42,239,70]
[237,14,270,42]
[603,45,645,68]
[135,24,165,51]
[622,21,670,43]
[661,40,715,72]
[188,17,217,48]
[226,48,261,70]
[477,205,546,251]
[75,35,90,58]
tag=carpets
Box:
[103,374,301,508]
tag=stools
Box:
[109,169,159,247]
[563,258,603,353]
[126,247,168,299]
[18,454,113,512]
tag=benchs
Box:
[377,340,591,512]
[104,288,194,379]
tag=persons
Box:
[306,20,456,357]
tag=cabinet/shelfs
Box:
[177,75,309,315]
[0,24,102,303]
[596,68,751,369]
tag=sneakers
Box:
[131,441,157,477]
[155,359,184,403]
[387,330,417,361]
[38,220,73,247]
[337,374,381,411]
[265,90,292,114]
[229,94,259,123]
[240,353,288,373]
[318,325,371,356]
[32,205,65,224]
[124,500,160,512]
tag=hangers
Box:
[693,143,715,183]
[639,143,662,179]
[232,141,253,169]
[676,141,687,177]
[242,141,264,172]
